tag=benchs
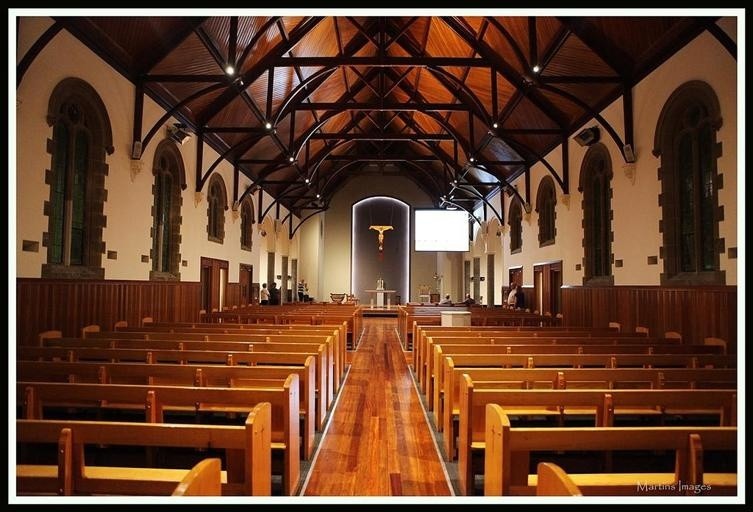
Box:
[15,302,362,500]
[395,302,724,498]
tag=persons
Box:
[439,294,451,304]
[298,280,309,302]
[508,283,525,309]
[261,283,278,305]
[464,294,474,302]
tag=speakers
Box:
[232,201,239,210]
[623,144,635,162]
[133,142,143,159]
[524,202,531,213]
[274,218,281,232]
